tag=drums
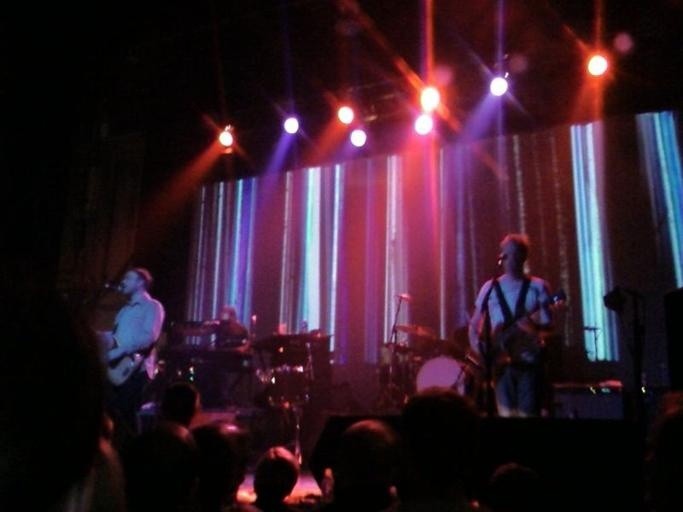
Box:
[416,355,475,403]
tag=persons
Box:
[212,306,251,349]
[95,266,168,445]
[465,232,557,421]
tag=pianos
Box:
[159,347,254,373]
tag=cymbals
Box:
[395,324,438,340]
[384,344,423,360]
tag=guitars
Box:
[89,328,134,386]
[468,292,567,380]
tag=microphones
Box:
[396,292,413,304]
[104,282,123,293]
[497,251,507,262]
